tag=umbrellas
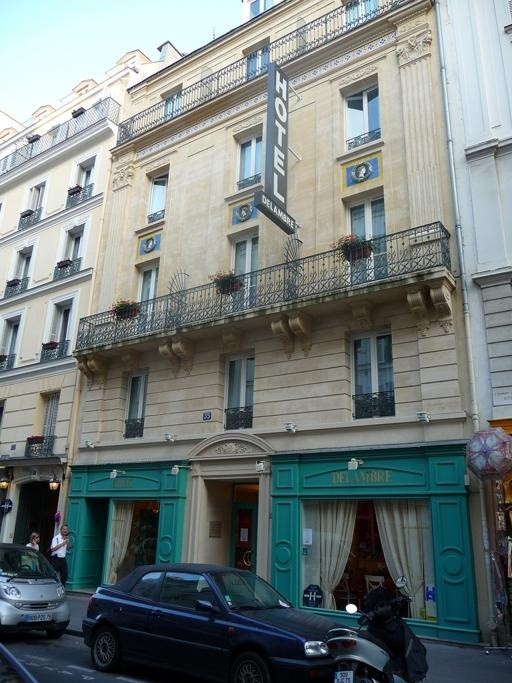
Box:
[466,427,512,481]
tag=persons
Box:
[49,525,69,587]
[26,532,42,573]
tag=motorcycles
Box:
[325,575,428,682]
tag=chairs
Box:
[334,574,361,611]
[365,574,385,593]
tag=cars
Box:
[82,564,353,683]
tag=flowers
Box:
[330,231,379,265]
[109,296,142,321]
[208,269,246,293]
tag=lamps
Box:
[348,456,364,471]
[110,468,127,478]
[48,463,65,494]
[255,459,266,472]
[0,466,15,490]
[171,464,193,475]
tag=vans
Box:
[0,542,71,641]
